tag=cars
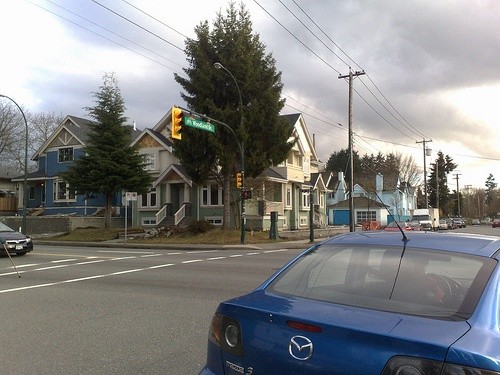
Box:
[198,229,500,375]
[383,207,500,231]
[0,220,34,257]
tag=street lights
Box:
[0,94,28,235]
[214,60,246,244]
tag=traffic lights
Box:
[237,173,243,189]
[172,107,184,140]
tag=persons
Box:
[432,218,437,231]
